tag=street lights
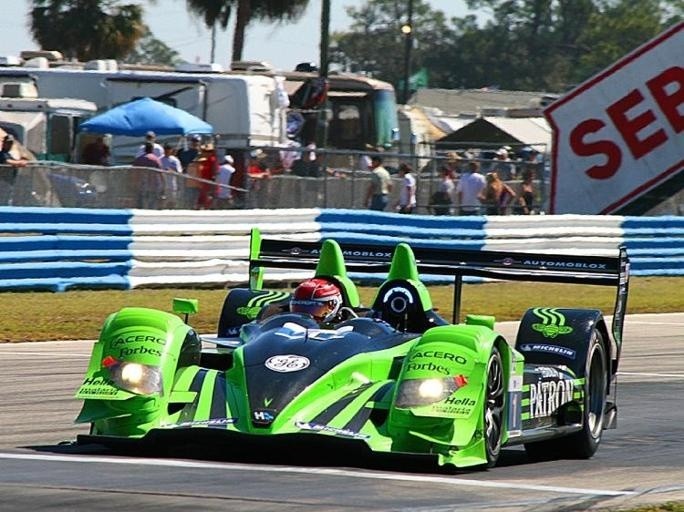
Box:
[401,0,413,104]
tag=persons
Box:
[245,148,272,209]
[289,143,347,209]
[358,144,392,211]
[426,146,539,215]
[81,133,114,190]
[129,132,236,210]
[391,163,417,213]
[0,134,27,206]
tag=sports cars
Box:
[74,227,631,475]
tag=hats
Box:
[200,143,264,165]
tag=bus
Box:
[227,55,400,172]
